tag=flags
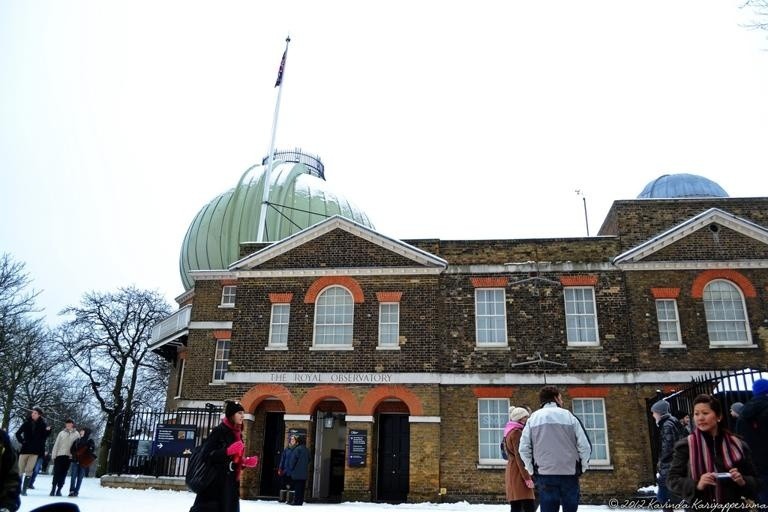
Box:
[273,48,286,85]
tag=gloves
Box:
[242,455,258,468]
[227,440,244,456]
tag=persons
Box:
[276,435,298,505]
[13,406,55,496]
[48,418,79,495]
[189,401,257,511]
[288,434,310,505]
[517,386,592,511]
[649,376,768,510]
[66,427,95,496]
[498,405,540,512]
[0,427,21,512]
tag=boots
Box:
[18,474,31,496]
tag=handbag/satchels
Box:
[500,437,509,461]
[186,439,207,495]
[78,453,97,468]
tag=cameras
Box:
[713,473,733,478]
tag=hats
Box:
[752,378,768,395]
[224,399,244,418]
[731,402,745,414]
[509,406,529,421]
[651,400,670,414]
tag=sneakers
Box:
[49,490,79,496]
[28,484,35,490]
